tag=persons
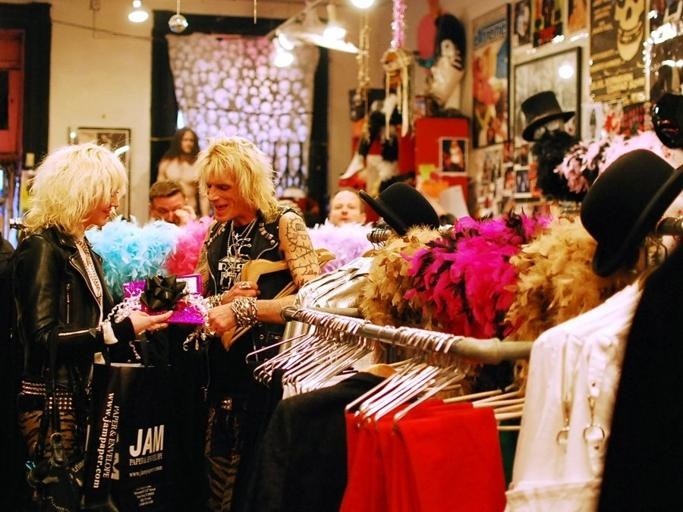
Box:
[147,181,195,226]
[157,127,202,216]
[188,136,321,511]
[339,11,467,181]
[1,141,174,512]
[328,187,368,226]
[448,139,463,171]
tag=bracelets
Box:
[208,293,224,308]
[229,296,258,328]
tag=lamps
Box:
[128,0,149,24]
[169,1,187,33]
[265,0,378,68]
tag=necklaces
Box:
[75,238,100,291]
[225,218,257,278]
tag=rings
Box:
[239,281,252,289]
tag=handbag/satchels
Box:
[21,448,84,512]
[80,358,175,512]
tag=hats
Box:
[579,147,683,278]
[518,89,577,141]
[356,180,441,241]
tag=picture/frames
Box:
[67,128,131,218]
[515,45,582,149]
[438,137,469,177]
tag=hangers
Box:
[368,227,392,252]
[245,305,526,433]
